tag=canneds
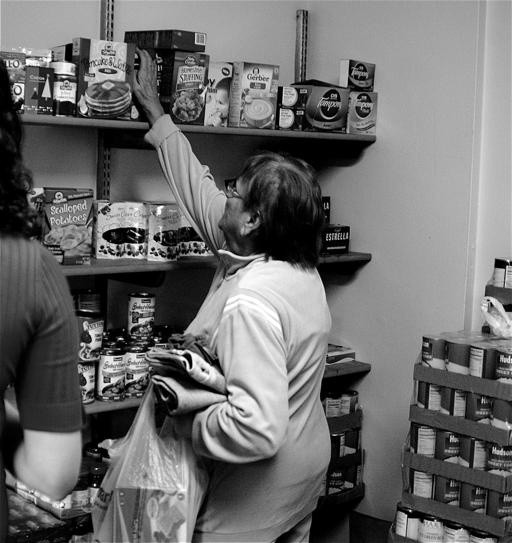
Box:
[69,256,366,543]
[388,258,512,542]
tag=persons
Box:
[129,41,336,543]
[0,56,85,542]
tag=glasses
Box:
[224,179,244,199]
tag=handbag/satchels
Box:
[92,383,210,543]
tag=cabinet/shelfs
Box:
[5,108,376,539]
[388,277,512,543]
